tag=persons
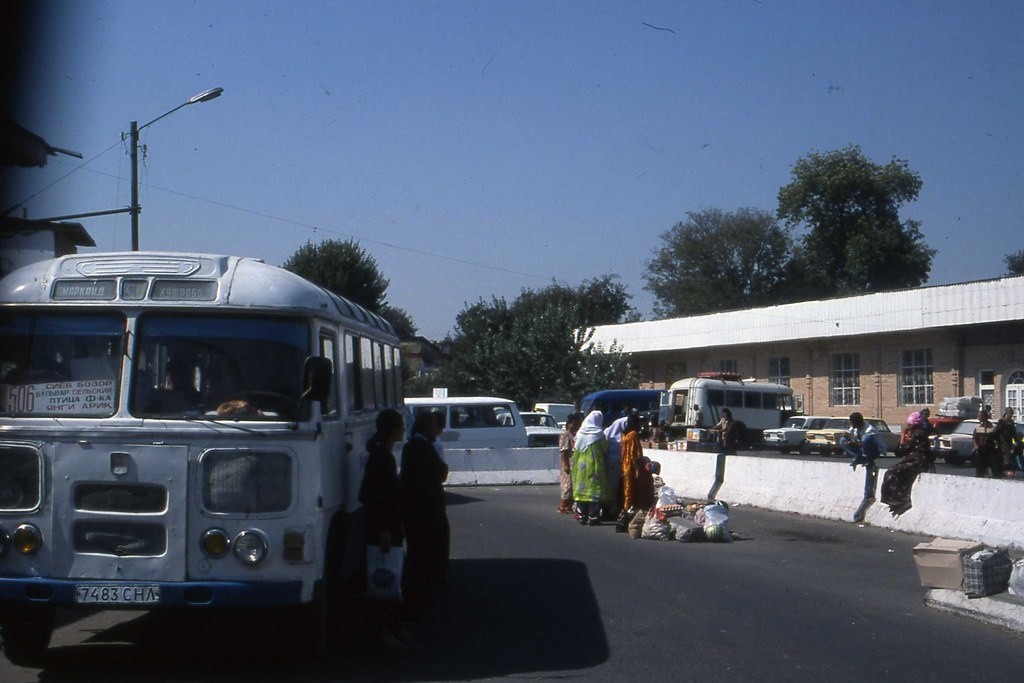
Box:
[839,412,886,466]
[711,408,736,455]
[357,409,450,644]
[972,405,1024,480]
[693,404,703,427]
[557,410,658,530]
[622,403,658,436]
[882,409,939,506]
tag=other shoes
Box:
[850,458,863,466]
[557,507,574,514]
[616,526,626,531]
[579,516,587,525]
[863,460,874,467]
[587,518,600,526]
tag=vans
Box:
[659,371,796,450]
[579,387,669,430]
[404,396,530,452]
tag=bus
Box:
[0,251,408,660]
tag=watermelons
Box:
[705,525,723,542]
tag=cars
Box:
[924,419,1024,465]
[494,412,562,448]
[803,416,902,457]
[760,416,830,455]
[895,415,968,459]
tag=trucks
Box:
[533,403,577,431]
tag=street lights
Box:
[128,85,228,256]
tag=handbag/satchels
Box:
[963,546,1012,599]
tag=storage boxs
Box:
[911,537,984,591]
[641,428,722,453]
